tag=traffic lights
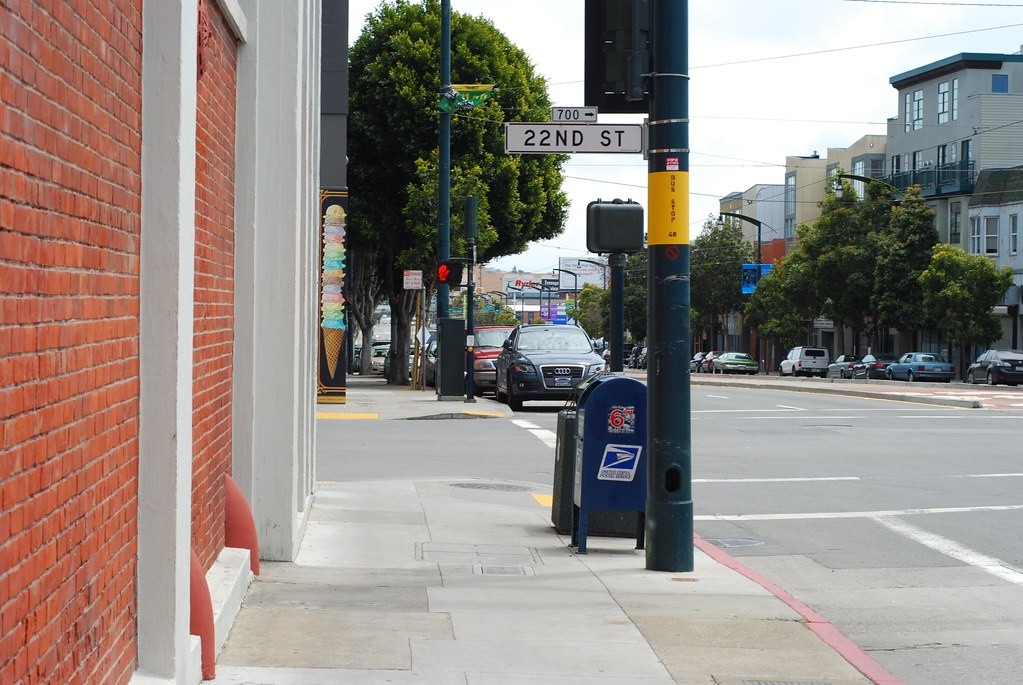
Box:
[436,259,463,285]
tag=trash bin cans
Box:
[552,373,638,537]
[570,377,648,555]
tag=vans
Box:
[779,346,829,378]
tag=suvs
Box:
[495,323,611,411]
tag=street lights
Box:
[473,290,508,315]
[552,269,577,325]
[577,259,606,351]
[835,174,918,352]
[717,212,761,374]
[529,283,551,320]
[521,285,542,318]
[505,283,523,327]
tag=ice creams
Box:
[321,205,347,378]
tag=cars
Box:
[601,343,647,369]
[852,354,899,379]
[966,349,1023,385]
[690,350,760,375]
[353,325,517,396]
[826,354,864,379]
[885,352,956,383]
